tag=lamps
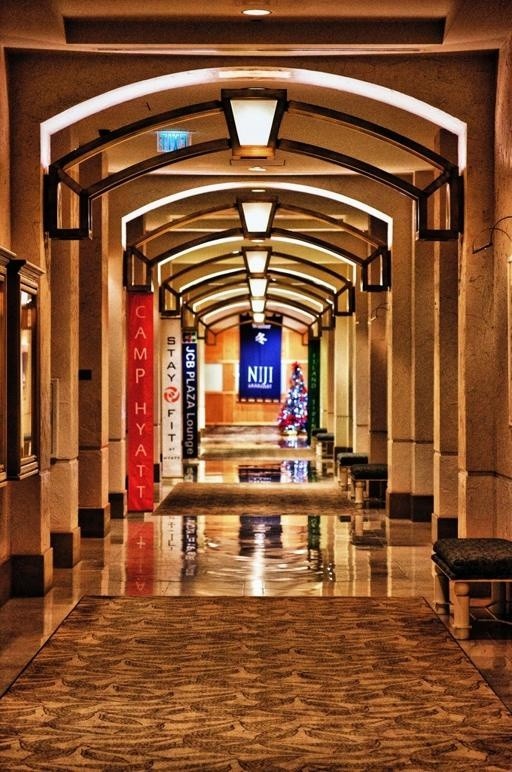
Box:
[216,84,290,162]
[217,190,289,298]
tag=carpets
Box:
[150,480,357,522]
[199,446,315,463]
[0,593,512,772]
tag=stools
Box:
[311,427,388,509]
[428,539,512,641]
